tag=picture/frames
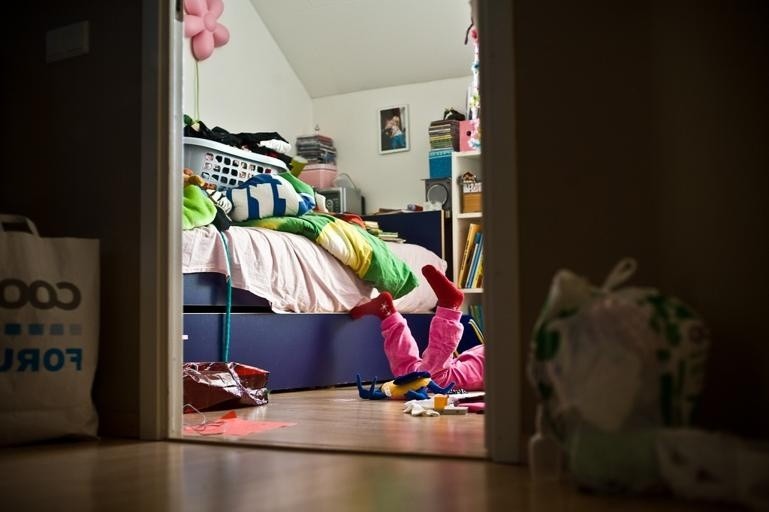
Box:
[378,105,408,154]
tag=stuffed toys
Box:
[353,372,457,403]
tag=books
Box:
[294,135,337,166]
[428,118,461,150]
[456,223,487,343]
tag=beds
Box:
[183,209,444,404]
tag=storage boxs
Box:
[293,163,338,189]
[462,181,482,214]
[458,120,481,152]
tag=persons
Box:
[381,113,404,150]
[343,264,485,394]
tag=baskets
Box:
[184,137,288,188]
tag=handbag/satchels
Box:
[183,362,270,413]
[0,228,100,447]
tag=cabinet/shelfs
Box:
[449,150,484,332]
